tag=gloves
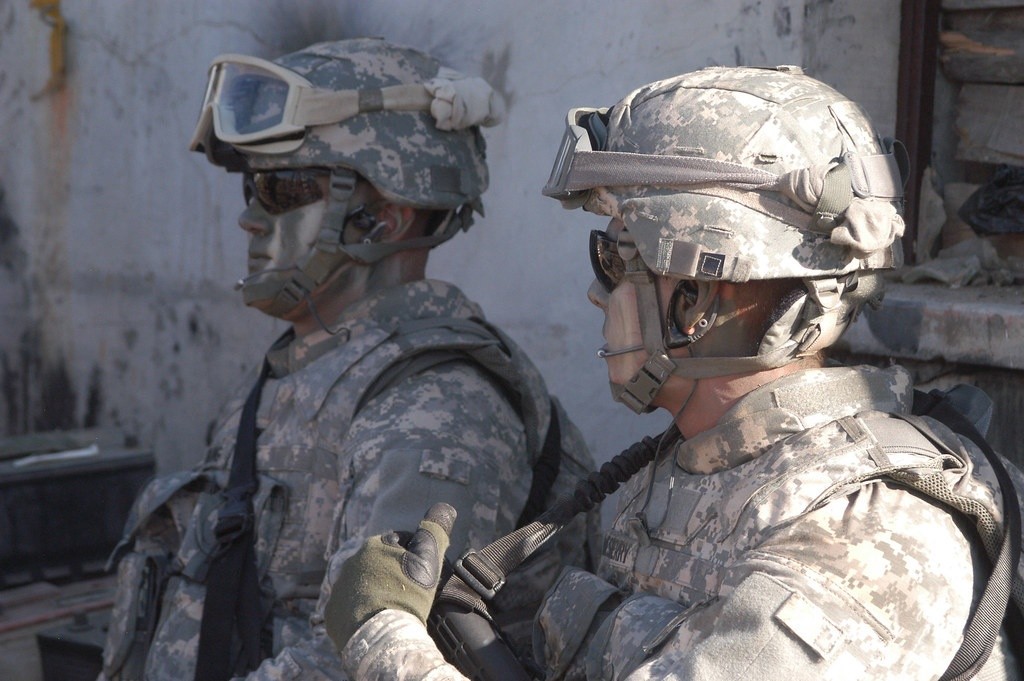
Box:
[316,498,460,650]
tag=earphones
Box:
[681,280,698,308]
[357,204,379,233]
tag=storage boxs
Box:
[0,445,159,583]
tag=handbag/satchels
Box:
[101,471,257,681]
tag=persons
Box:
[99,39,600,680]
[323,65,1024,681]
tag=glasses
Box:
[538,108,905,258]
[238,170,331,208]
[585,227,644,295]
[182,55,505,148]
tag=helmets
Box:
[227,38,491,212]
[599,63,909,282]
